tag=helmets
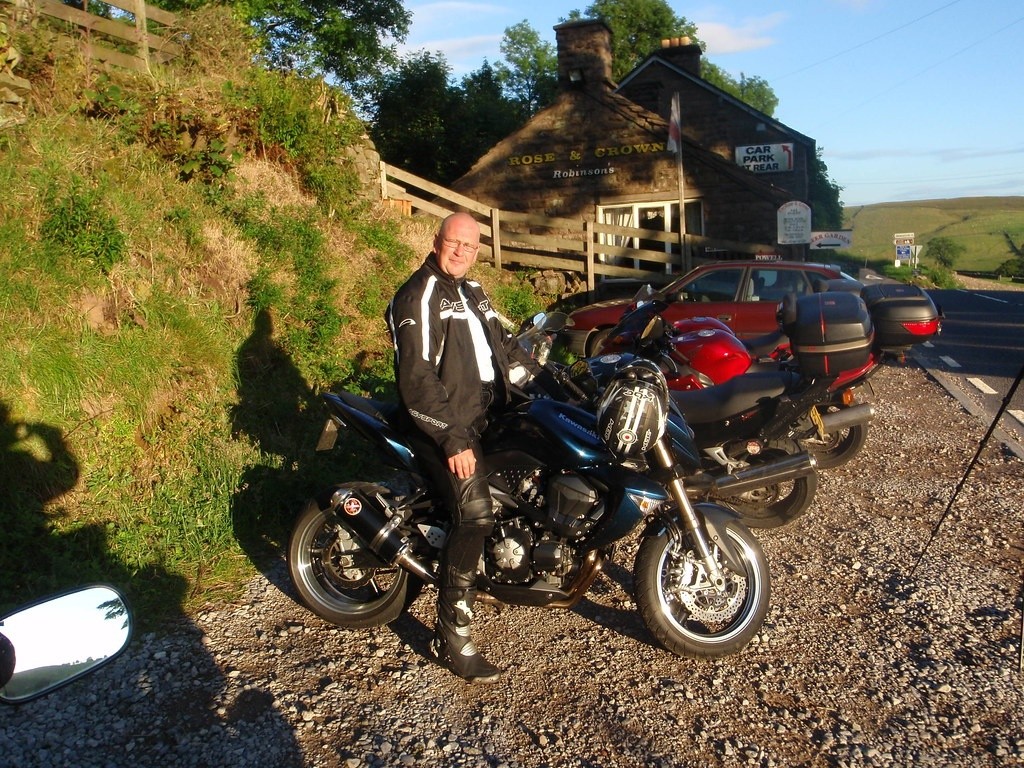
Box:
[594,358,670,459]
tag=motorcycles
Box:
[500,272,944,534]
[285,280,776,660]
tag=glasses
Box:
[439,234,478,253]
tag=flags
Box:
[666,95,680,154]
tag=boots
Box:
[424,583,501,685]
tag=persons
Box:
[387,211,581,684]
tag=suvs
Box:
[565,258,885,389]
